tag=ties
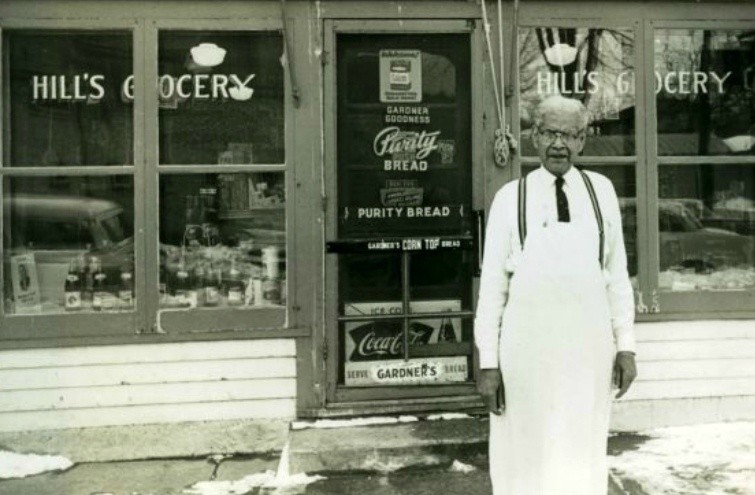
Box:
[553,178,573,223]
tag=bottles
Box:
[64,254,281,311]
[438,306,455,342]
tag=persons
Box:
[19,265,30,291]
[473,95,637,495]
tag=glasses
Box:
[538,127,584,146]
